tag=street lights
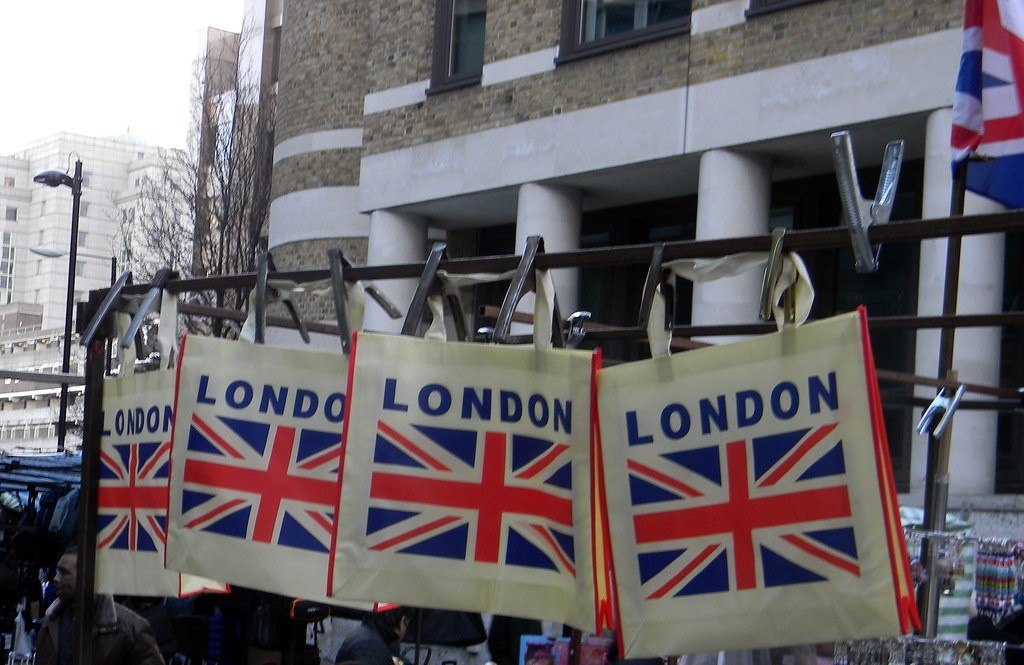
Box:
[29,245,117,376]
[33,152,83,453]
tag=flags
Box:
[948,0,1023,215]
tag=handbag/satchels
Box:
[248,646,282,665]
[305,645,320,665]
[392,646,432,665]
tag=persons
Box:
[0,544,165,665]
[335,602,412,665]
